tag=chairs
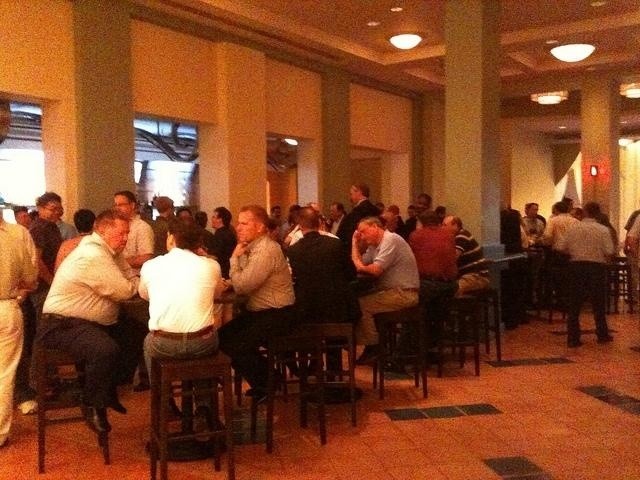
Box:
[34,342,110,474]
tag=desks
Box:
[120,289,243,331]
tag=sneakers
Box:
[18,401,39,414]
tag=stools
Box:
[150,350,236,480]
[373,289,501,399]
[251,323,356,453]
[529,246,634,322]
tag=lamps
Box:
[385,29,427,51]
[530,90,572,106]
[615,76,640,100]
[542,39,602,63]
[589,164,599,177]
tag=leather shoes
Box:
[567,337,613,347]
[355,346,390,365]
[78,396,111,433]
[97,391,126,414]
[168,389,269,443]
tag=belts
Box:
[153,324,213,340]
[41,312,64,321]
